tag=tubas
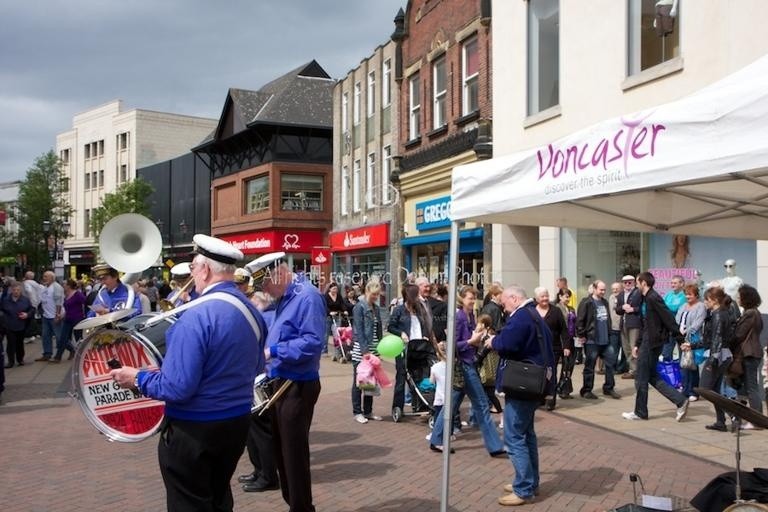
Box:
[73,213,163,340]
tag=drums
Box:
[70,312,178,443]
[251,373,272,414]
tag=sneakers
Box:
[411,388,505,441]
[23,336,36,344]
[354,415,369,424]
[740,422,764,430]
[368,415,384,421]
[676,399,690,422]
[622,412,643,420]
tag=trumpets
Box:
[160,278,194,312]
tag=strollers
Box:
[392,338,442,429]
[331,311,352,363]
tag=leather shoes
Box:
[430,444,455,455]
[238,473,256,484]
[243,481,280,493]
[498,493,535,507]
[490,448,507,458]
[705,422,728,432]
[503,484,515,493]
[732,417,742,433]
[4,347,78,369]
[575,358,635,380]
[547,390,622,411]
[689,396,698,403]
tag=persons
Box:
[244,251,327,512]
[667,234,695,268]
[0,262,763,441]
[238,291,281,492]
[430,285,506,457]
[108,233,268,512]
[484,283,555,507]
[720,259,745,315]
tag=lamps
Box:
[178,220,191,241]
[156,218,166,235]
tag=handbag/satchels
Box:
[356,354,393,397]
[657,358,682,390]
[689,328,707,365]
[502,359,548,399]
[726,352,745,380]
[453,362,465,392]
[680,332,698,371]
[557,358,573,395]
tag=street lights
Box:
[42,219,70,270]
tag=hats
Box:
[192,233,244,265]
[170,262,191,280]
[622,275,635,281]
[92,263,115,277]
[244,251,288,287]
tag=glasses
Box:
[625,282,631,284]
[724,265,733,267]
[189,263,198,271]
